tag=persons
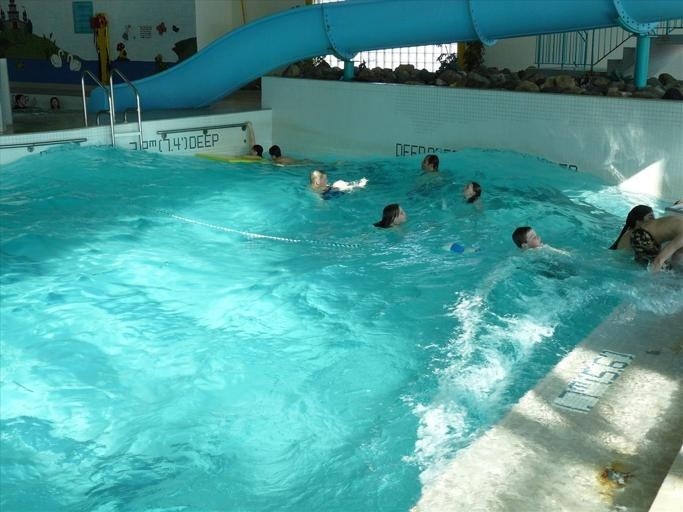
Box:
[416,153,440,181]
[267,144,342,168]
[307,170,358,203]
[461,181,482,204]
[511,224,573,258]
[13,93,37,109]
[606,203,656,249]
[372,201,408,233]
[618,213,682,277]
[243,121,265,160]
[47,97,61,113]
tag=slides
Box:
[85,0,681,114]
